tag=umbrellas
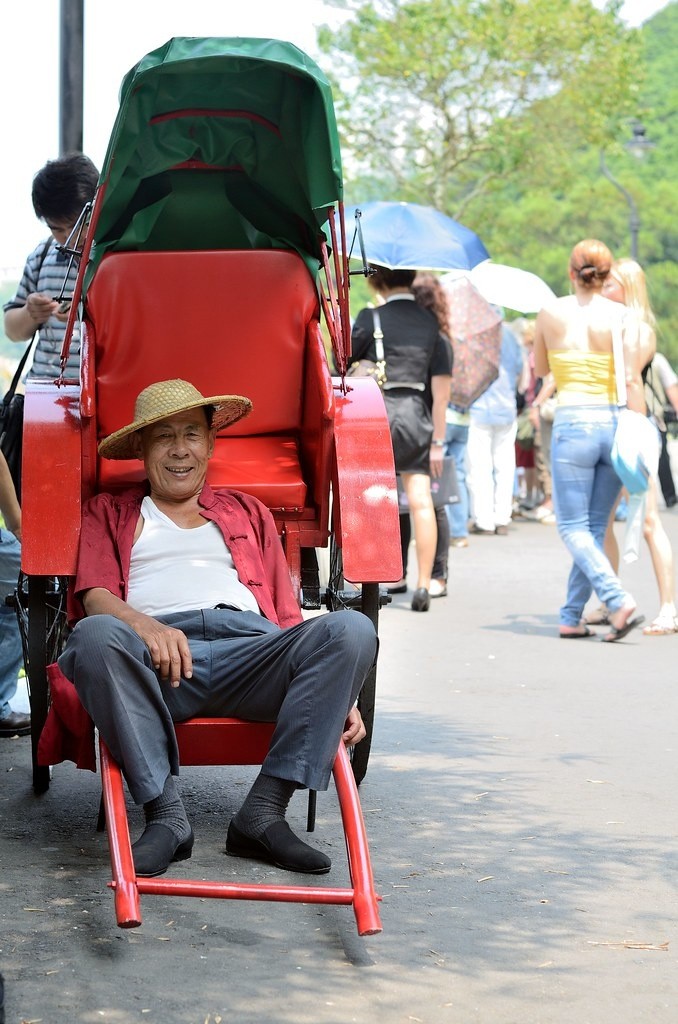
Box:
[327,200,491,270]
[454,264,557,313]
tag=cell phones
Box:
[59,300,72,314]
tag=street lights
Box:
[599,118,652,261]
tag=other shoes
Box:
[644,613,678,634]
[431,583,447,597]
[665,495,678,508]
[580,607,608,624]
[448,535,467,549]
[224,818,333,873]
[130,822,195,876]
[468,521,507,534]
[614,502,630,520]
[389,583,408,594]
[522,505,556,523]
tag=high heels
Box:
[411,590,430,612]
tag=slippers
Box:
[602,614,646,640]
[560,623,596,639]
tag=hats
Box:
[97,378,253,460]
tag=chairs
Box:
[78,249,333,511]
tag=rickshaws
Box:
[5,35,404,936]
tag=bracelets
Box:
[431,439,447,446]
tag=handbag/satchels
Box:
[345,311,387,394]
[0,391,24,492]
[607,408,662,493]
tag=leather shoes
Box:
[0,711,31,737]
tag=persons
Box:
[535,239,677,641]
[0,448,31,736]
[57,379,380,878]
[3,154,100,384]
[387,275,550,598]
[331,263,454,610]
